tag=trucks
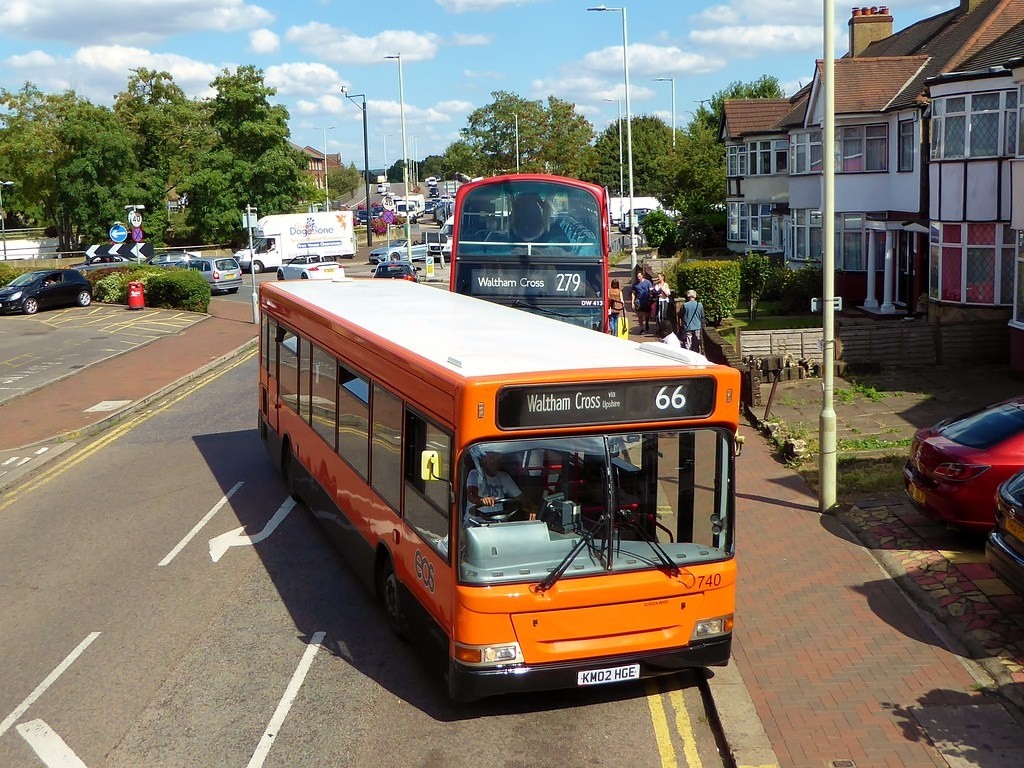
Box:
[234,211,358,275]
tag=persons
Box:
[657,320,681,348]
[266,240,275,253]
[651,273,671,336]
[675,289,705,355]
[608,279,626,337]
[631,271,653,335]
[463,451,537,529]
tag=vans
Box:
[173,257,243,295]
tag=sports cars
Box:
[368,238,428,265]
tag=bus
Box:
[448,173,626,333]
[256,276,745,700]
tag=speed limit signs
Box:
[131,213,143,227]
[383,197,395,212]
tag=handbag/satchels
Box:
[680,328,686,341]
[650,301,657,318]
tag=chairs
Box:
[458,234,486,255]
[486,234,512,255]
[560,215,600,256]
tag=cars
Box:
[984,468,1024,596]
[69,255,138,276]
[143,251,202,268]
[0,269,93,316]
[616,207,675,234]
[900,396,1024,540]
[354,175,460,261]
[369,261,422,284]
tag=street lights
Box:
[585,5,640,282]
[600,99,625,222]
[313,125,337,211]
[508,111,521,175]
[652,77,679,149]
[339,85,373,246]
[0,181,15,260]
[382,51,413,263]
[375,133,393,181]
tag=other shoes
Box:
[645,322,649,331]
[639,330,644,334]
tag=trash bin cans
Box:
[127,282,145,310]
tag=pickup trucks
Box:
[276,254,346,283]
